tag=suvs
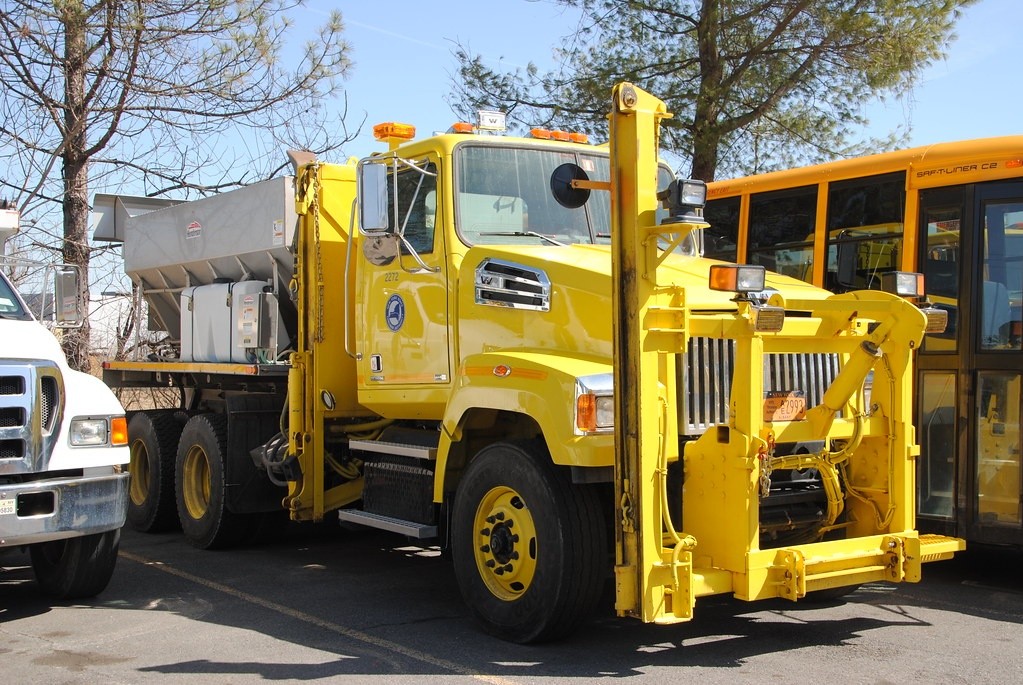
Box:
[0,263,130,603]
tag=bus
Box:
[703,136,1023,562]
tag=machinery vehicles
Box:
[91,82,967,644]
[704,218,1023,353]
